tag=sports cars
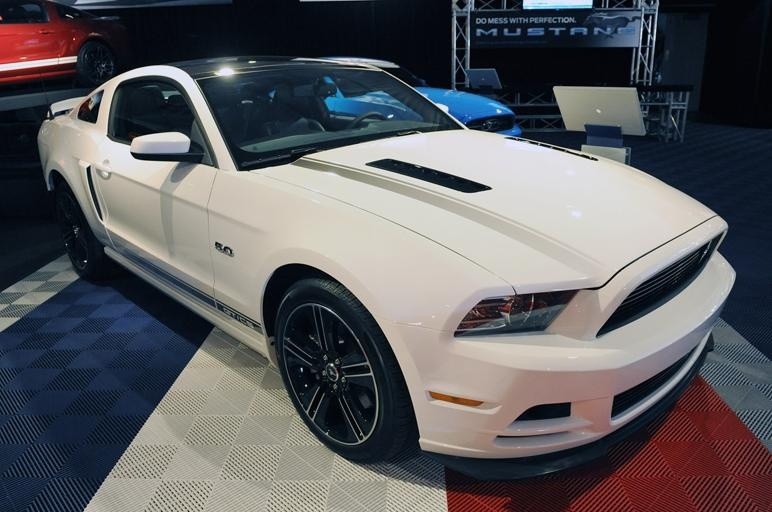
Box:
[1,0,127,87]
[37,56,736,480]
[268,59,523,139]
